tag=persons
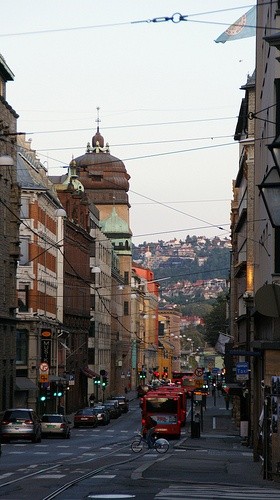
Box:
[142,411,155,449]
[89,393,95,407]
[224,394,230,410]
[212,376,216,384]
[57,405,64,415]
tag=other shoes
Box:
[147,446,152,449]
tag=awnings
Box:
[15,377,39,390]
[81,368,99,378]
[48,374,66,381]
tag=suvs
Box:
[0,409,42,443]
[40,413,71,439]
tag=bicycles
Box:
[131,430,170,454]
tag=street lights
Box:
[203,384,208,411]
[95,376,108,405]
[140,374,145,389]
[53,391,62,414]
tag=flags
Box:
[214,332,230,354]
[216,6,257,43]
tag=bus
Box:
[138,372,225,440]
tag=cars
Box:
[74,396,129,428]
